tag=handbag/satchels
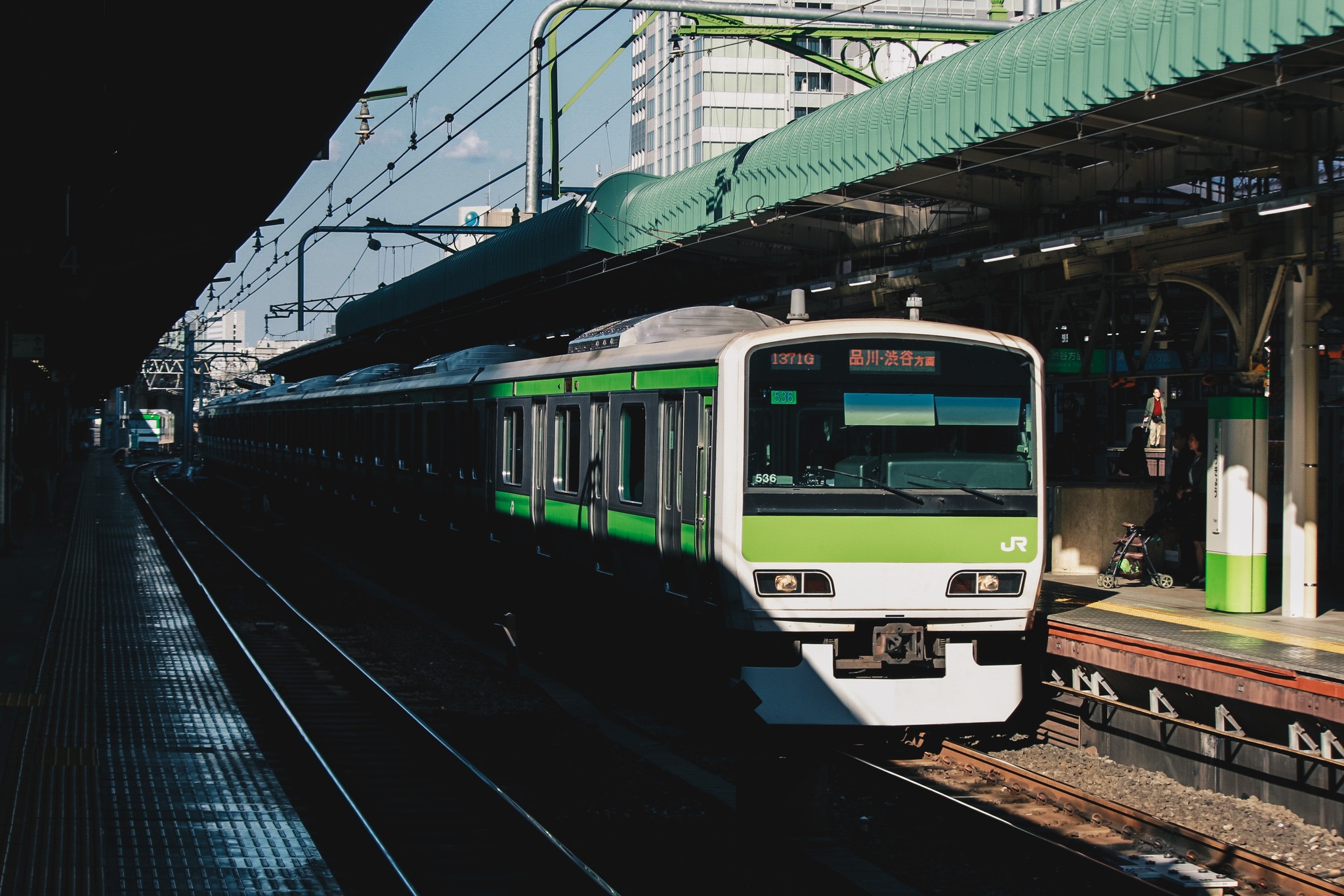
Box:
[1152,416,1163,424]
[1119,447,1139,474]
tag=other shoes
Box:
[1186,575,1205,587]
[1150,444,1155,448]
[1155,446,1159,448]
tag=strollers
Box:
[1097,490,1191,589]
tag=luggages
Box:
[1129,419,1150,448]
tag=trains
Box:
[196,305,1050,753]
[128,408,174,457]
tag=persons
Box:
[1144,388,1166,449]
[1168,431,1207,586]
